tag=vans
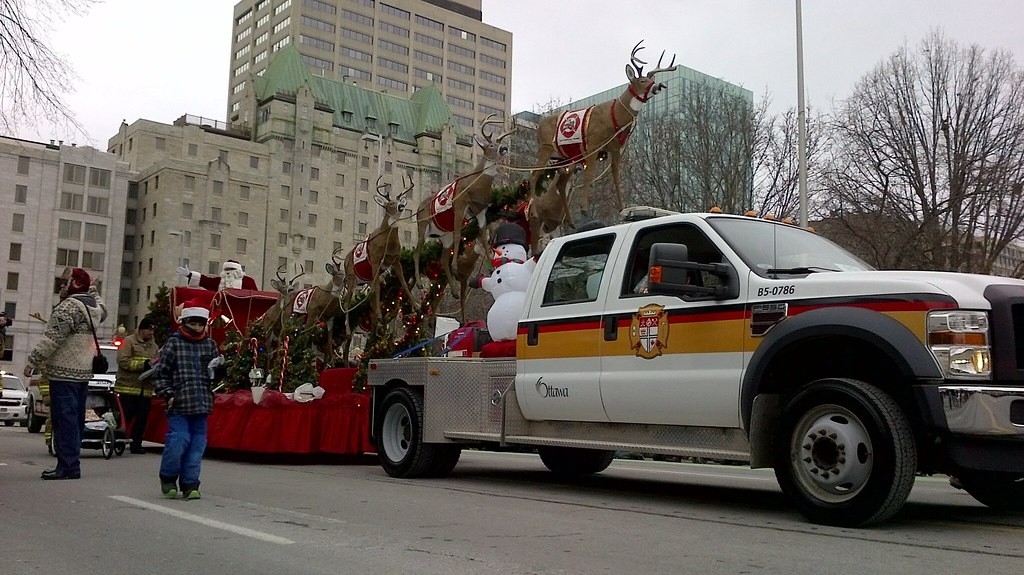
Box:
[0,371,32,427]
[26,341,116,425]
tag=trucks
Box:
[367,212,1024,527]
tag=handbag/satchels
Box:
[93,354,111,374]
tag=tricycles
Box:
[43,378,131,459]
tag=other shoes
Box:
[183,490,201,499]
[162,478,178,497]
[131,447,145,454]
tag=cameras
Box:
[203,355,214,364]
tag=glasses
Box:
[59,280,68,286]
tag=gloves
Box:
[176,264,190,276]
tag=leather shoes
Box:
[40,470,80,479]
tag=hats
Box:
[223,259,242,270]
[177,291,213,321]
[57,267,90,296]
[139,317,157,328]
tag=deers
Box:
[255,38,677,370]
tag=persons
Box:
[114,317,160,456]
[176,259,258,291]
[151,292,228,499]
[0,311,12,398]
[23,267,107,481]
[37,365,53,453]
